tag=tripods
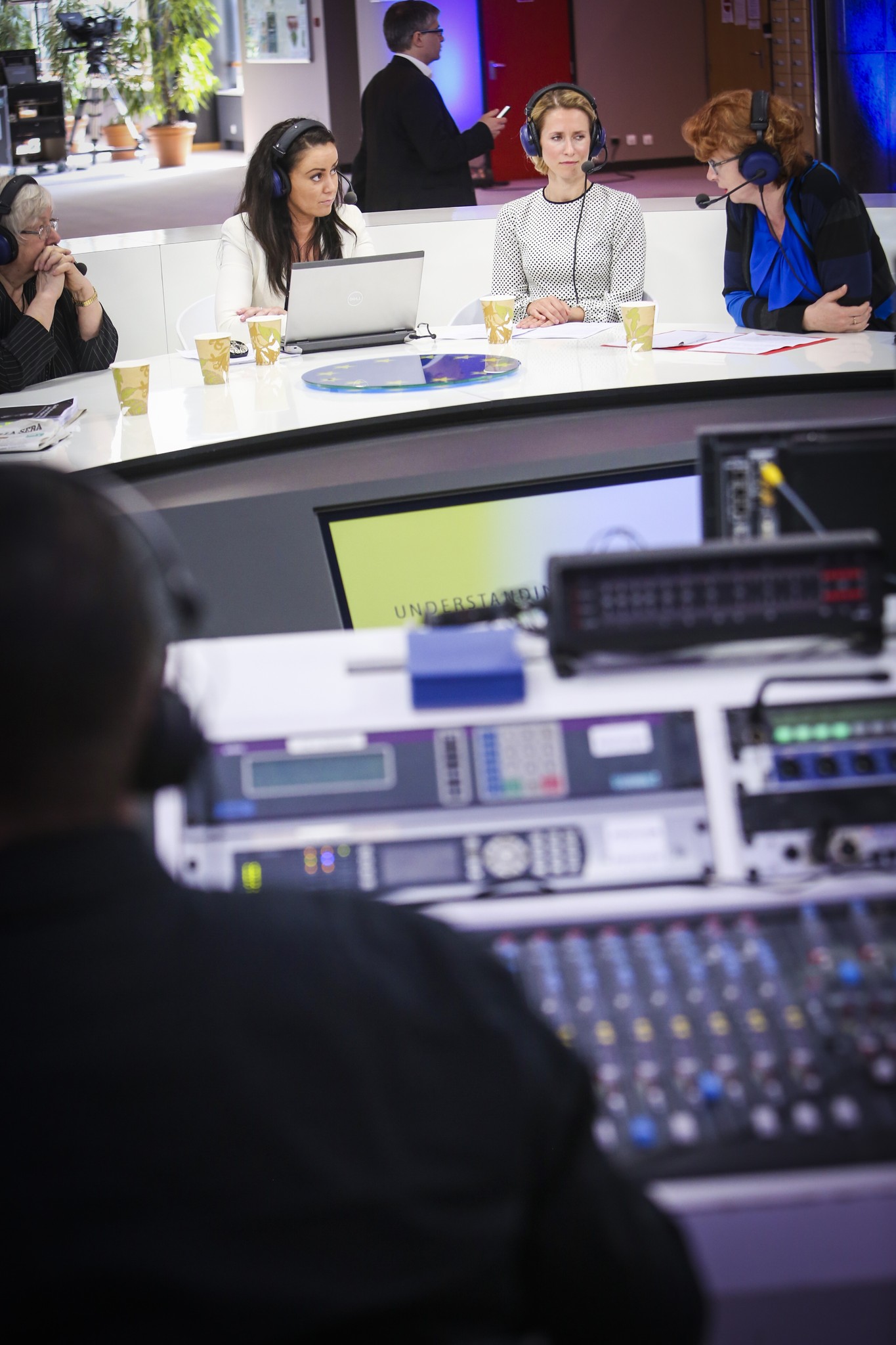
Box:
[64,61,144,153]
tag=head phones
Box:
[271,120,326,200]
[0,175,35,265]
[520,83,606,159]
[739,90,782,185]
[72,463,215,798]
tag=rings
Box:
[853,316,856,325]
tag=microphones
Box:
[53,243,87,276]
[696,169,768,209]
[335,170,356,205]
[581,145,608,174]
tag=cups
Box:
[111,361,150,416]
[479,295,517,344]
[619,301,657,352]
[193,332,231,385]
[246,315,282,365]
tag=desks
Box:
[0,323,896,635]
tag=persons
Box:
[215,117,369,346]
[492,87,646,330]
[343,0,506,213]
[0,174,118,395]
[680,90,896,334]
[0,462,710,1345]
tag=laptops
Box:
[280,251,425,356]
[3,64,45,87]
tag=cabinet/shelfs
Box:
[0,81,67,176]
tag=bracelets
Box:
[71,286,98,306]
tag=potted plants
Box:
[0,0,224,168]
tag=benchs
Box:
[63,196,735,366]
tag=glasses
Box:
[19,217,58,239]
[410,28,444,39]
[708,153,741,176]
